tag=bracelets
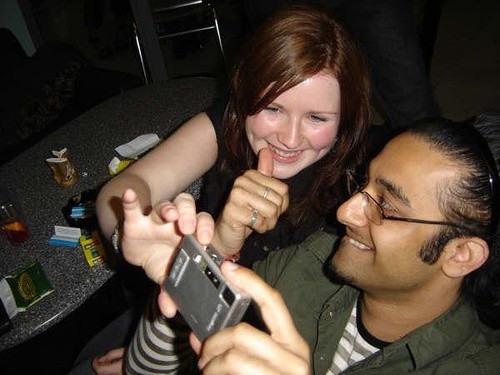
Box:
[222,251,241,263]
[111,218,126,254]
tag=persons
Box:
[70,9,376,375]
[122,114,500,375]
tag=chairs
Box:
[132,1,224,84]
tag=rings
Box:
[262,186,270,199]
[248,208,259,228]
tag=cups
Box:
[1,203,33,246]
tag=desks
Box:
[0,76,220,350]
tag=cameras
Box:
[161,234,251,345]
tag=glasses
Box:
[345,170,491,241]
